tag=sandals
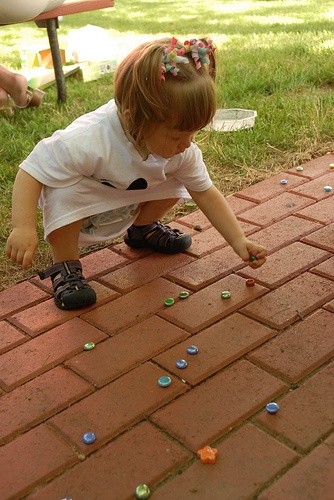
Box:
[37,259,98,309]
[123,219,192,253]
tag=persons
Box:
[0,0,67,117]
[4,38,268,311]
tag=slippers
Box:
[13,87,45,110]
[0,106,15,117]
